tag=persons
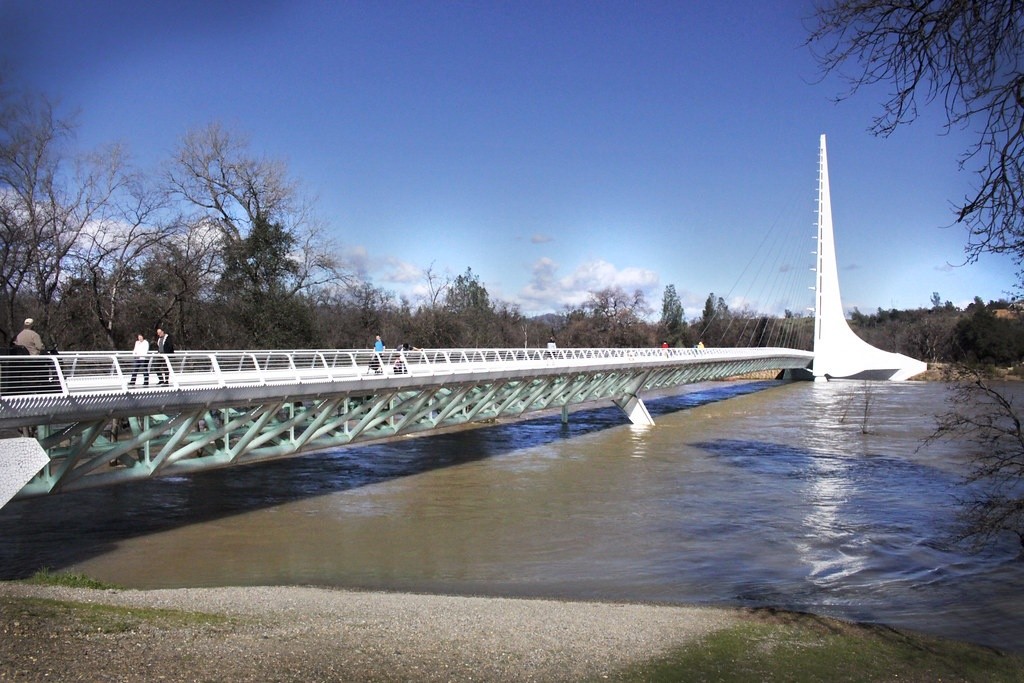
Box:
[661,340,669,348]
[395,342,423,374]
[127,333,150,387]
[373,334,385,373]
[14,318,49,362]
[546,336,556,359]
[698,342,704,349]
[154,329,175,386]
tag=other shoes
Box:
[157,381,168,387]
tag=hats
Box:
[24,318,34,326]
